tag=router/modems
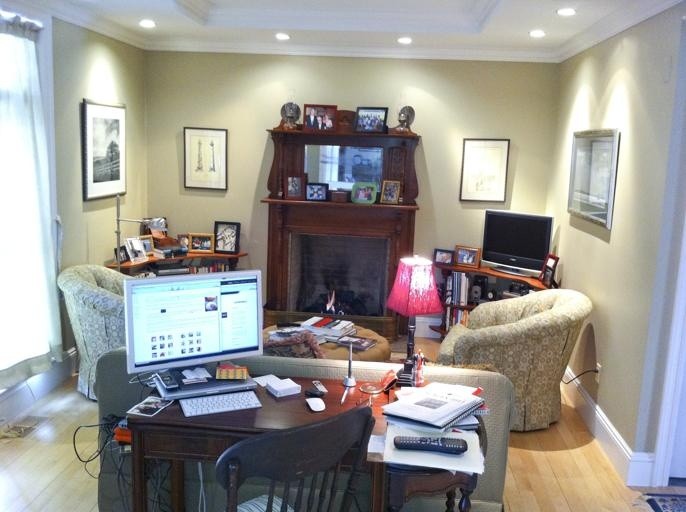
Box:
[265,378,302,398]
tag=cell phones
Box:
[155,371,179,391]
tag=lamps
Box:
[116,197,168,272]
[384,255,444,387]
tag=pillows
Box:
[264,331,325,360]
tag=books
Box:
[335,335,378,351]
[128,259,230,279]
[300,315,356,343]
[380,379,490,432]
[444,306,474,333]
[443,271,471,307]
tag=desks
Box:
[127,372,488,512]
[107,241,247,271]
[429,261,549,342]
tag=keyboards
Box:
[178,390,263,418]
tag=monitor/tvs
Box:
[480,208,553,278]
[123,269,265,401]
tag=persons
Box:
[316,116,326,130]
[306,109,317,128]
[323,113,332,128]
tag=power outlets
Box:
[594,363,603,384]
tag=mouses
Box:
[305,397,326,412]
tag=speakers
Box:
[471,275,489,303]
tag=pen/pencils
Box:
[415,349,425,385]
[348,341,352,379]
[340,387,348,405]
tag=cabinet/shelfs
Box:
[261,109,420,339]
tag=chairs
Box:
[216,405,376,512]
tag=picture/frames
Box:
[183,126,227,190]
[189,233,214,254]
[303,103,338,129]
[459,137,510,203]
[567,129,621,231]
[83,97,126,201]
[114,244,131,264]
[138,234,154,256]
[354,106,388,132]
[307,183,329,202]
[455,244,482,268]
[381,180,401,205]
[144,217,168,236]
[284,173,307,202]
[351,182,376,204]
[214,220,240,253]
[338,144,382,184]
[434,248,454,266]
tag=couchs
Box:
[98,347,513,511]
[57,263,155,403]
[438,289,593,433]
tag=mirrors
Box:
[304,144,383,192]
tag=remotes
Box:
[393,435,468,455]
[311,380,328,393]
